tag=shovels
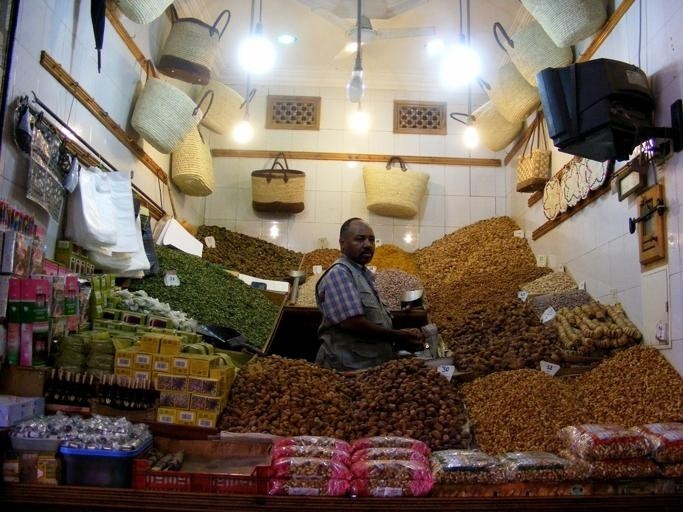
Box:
[287,269,307,304]
[195,322,265,359]
[400,288,426,311]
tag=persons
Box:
[315,218,426,372]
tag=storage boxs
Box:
[10,433,58,456]
[59,435,154,489]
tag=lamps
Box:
[233,0,481,150]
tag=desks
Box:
[0,476,682,512]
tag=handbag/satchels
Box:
[450,0,609,153]
[251,151,306,214]
[515,110,552,193]
[362,155,430,219]
[119,1,257,198]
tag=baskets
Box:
[131,437,275,496]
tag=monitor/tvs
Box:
[537,60,653,162]
